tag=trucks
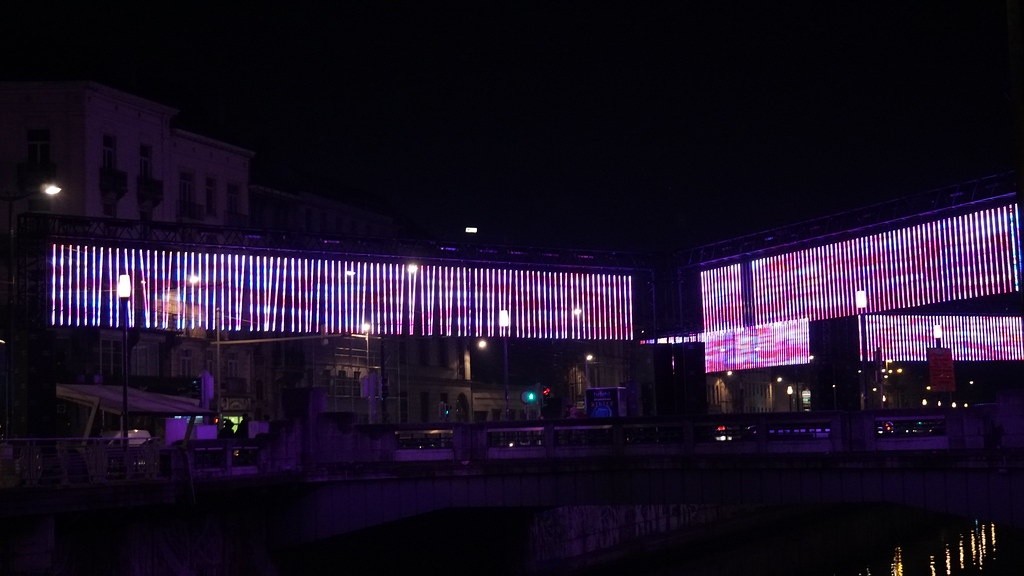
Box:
[585,386,629,421]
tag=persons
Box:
[218,414,270,438]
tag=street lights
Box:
[856,290,869,410]
[934,324,948,410]
[499,310,509,424]
[120,274,132,448]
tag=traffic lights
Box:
[528,390,537,400]
[541,386,549,397]
